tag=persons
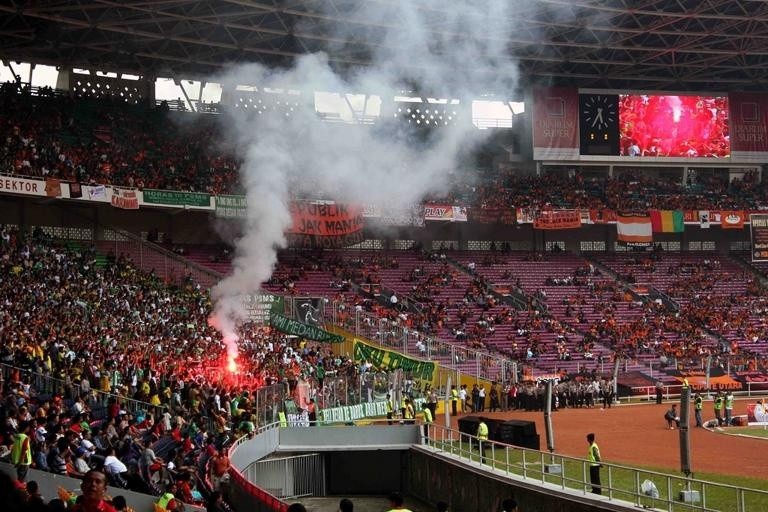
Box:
[1,78,768,213]
[1,227,439,511]
[656,378,664,404]
[409,240,768,376]
[682,376,688,387]
[587,433,603,495]
[694,390,734,428]
[477,417,489,464]
[450,376,617,416]
[664,404,679,429]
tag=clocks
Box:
[579,94,619,158]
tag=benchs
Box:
[105,240,768,404]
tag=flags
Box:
[617,210,685,242]
